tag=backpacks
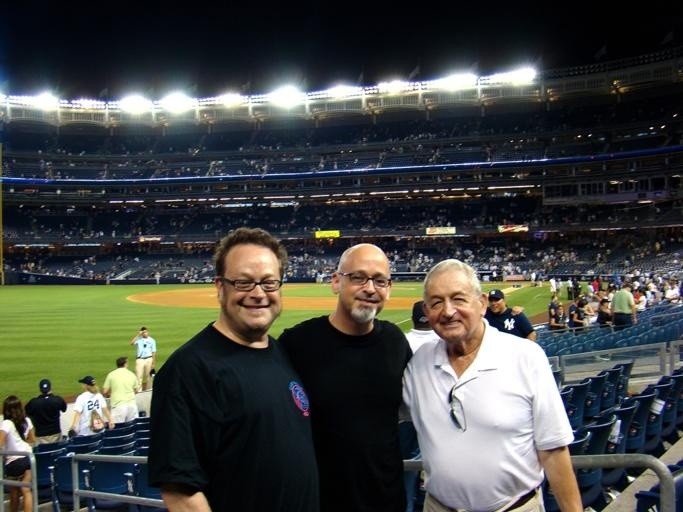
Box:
[90,410,105,432]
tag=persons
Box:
[24,379,67,445]
[277,241,414,512]
[147,227,321,512]
[129,326,157,394]
[70,375,116,434]
[401,259,585,512]
[0,395,37,512]
[102,356,144,424]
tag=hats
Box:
[600,299,611,303]
[413,301,429,323]
[78,376,96,386]
[39,380,50,392]
[489,290,504,299]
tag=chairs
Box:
[27,365,683,511]
[0,106,681,278]
[533,278,683,364]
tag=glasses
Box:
[224,278,282,291]
[449,385,466,432]
[339,271,391,286]
